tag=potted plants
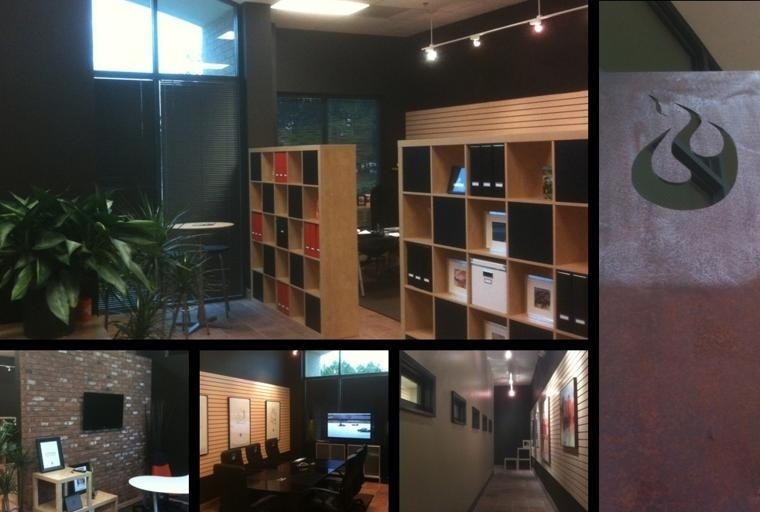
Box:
[0,188,160,340]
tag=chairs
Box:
[213,437,368,512]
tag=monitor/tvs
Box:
[326,411,373,444]
[82,391,125,434]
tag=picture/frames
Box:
[35,436,65,472]
[199,394,281,455]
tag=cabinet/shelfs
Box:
[32,466,119,512]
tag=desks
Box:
[168,220,236,336]
[128,473,190,511]
